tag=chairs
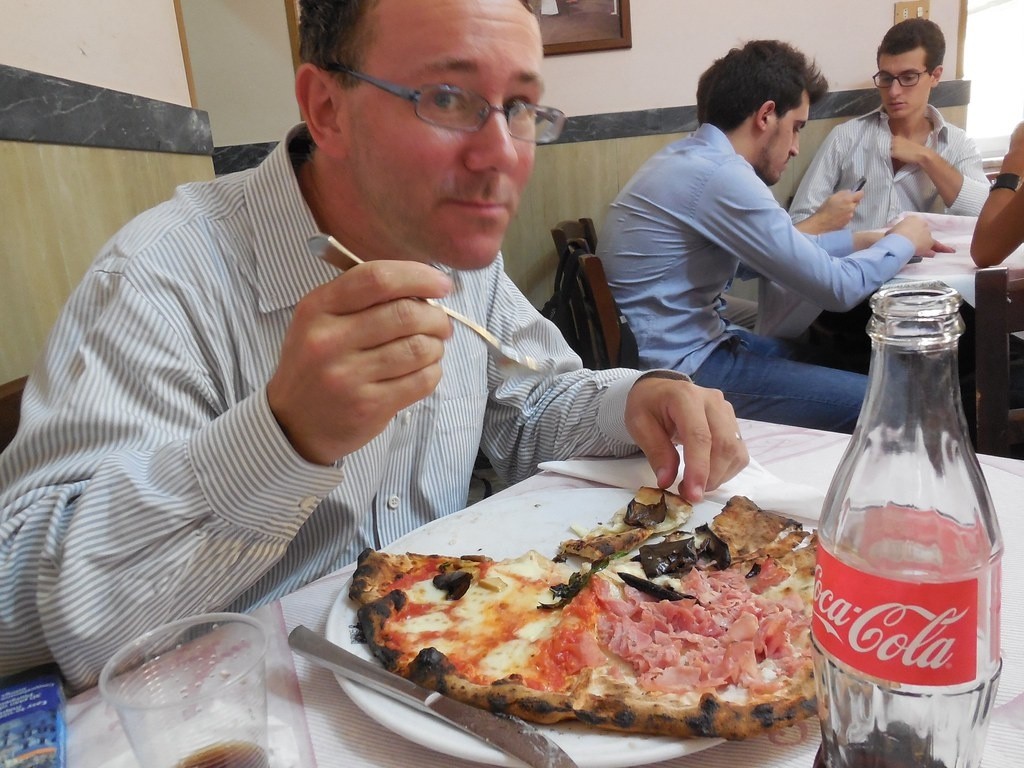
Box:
[550,215,621,369]
[974,266,1024,459]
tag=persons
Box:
[790,19,992,230]
[970,120,1024,268]
[597,40,955,434]
[0,0,749,694]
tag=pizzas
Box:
[349,485,818,743]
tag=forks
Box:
[304,233,581,380]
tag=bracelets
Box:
[990,173,1023,193]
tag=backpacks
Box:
[537,240,639,369]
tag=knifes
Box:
[287,624,580,768]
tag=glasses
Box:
[320,59,570,145]
[872,69,927,89]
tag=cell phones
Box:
[851,176,867,192]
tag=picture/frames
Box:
[525,0,632,54]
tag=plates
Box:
[328,489,732,768]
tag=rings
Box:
[734,432,742,440]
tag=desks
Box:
[805,280,974,369]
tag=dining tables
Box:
[60,416,1024,768]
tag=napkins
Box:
[536,443,826,521]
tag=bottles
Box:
[803,281,1005,768]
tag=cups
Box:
[98,611,270,768]
